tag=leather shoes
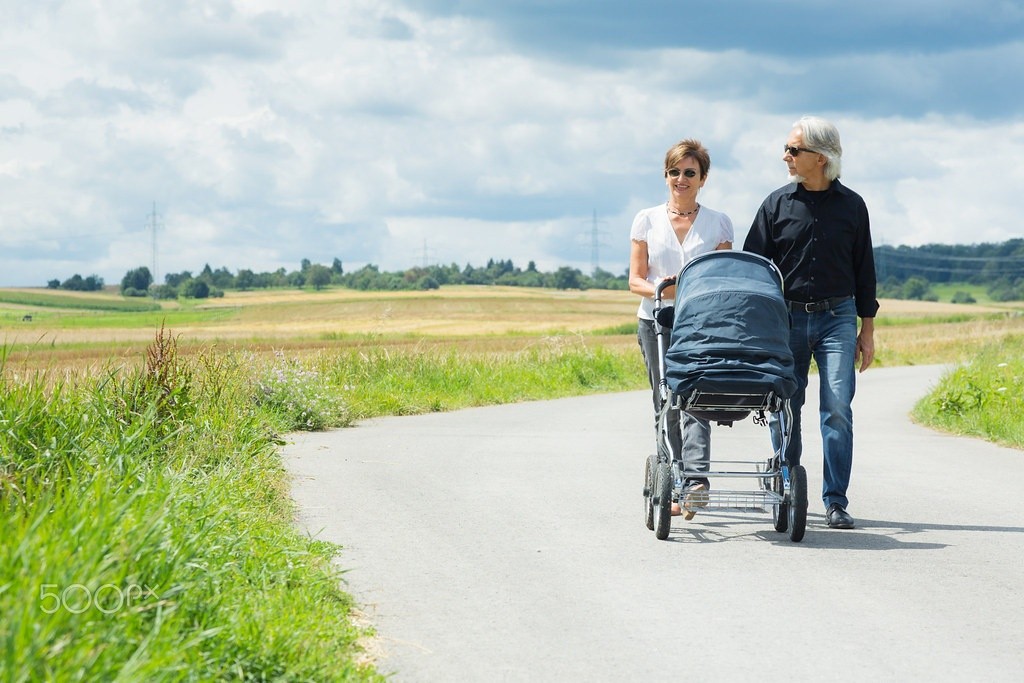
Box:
[825,503,855,528]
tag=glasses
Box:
[785,145,819,157]
[665,169,701,178]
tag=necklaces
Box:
[667,200,700,215]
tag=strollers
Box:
[642,249,809,543]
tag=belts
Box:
[788,296,850,313]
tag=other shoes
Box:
[670,491,682,515]
[681,484,709,520]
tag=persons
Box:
[742,116,879,529]
[629,139,734,521]
